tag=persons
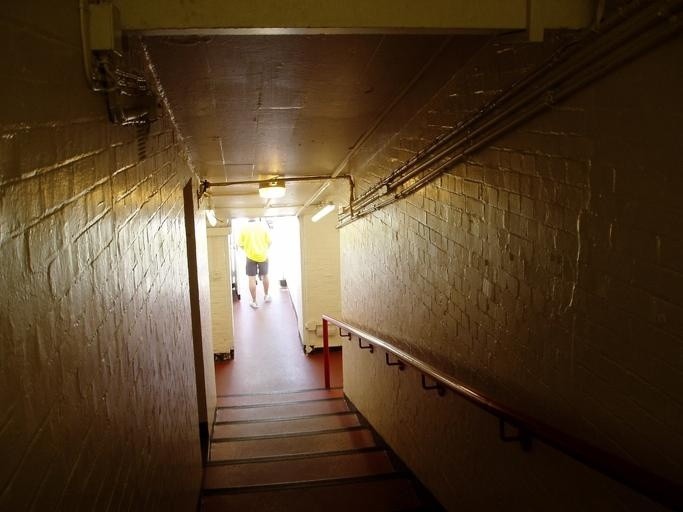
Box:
[254,217,273,285]
[239,217,272,309]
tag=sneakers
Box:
[264,295,272,302]
[249,302,259,309]
